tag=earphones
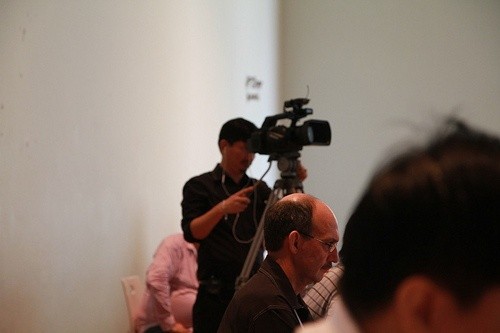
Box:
[224,146,228,155]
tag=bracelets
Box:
[223,200,228,219]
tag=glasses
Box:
[290,228,338,253]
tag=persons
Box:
[134,233,199,333]
[181,118,308,333]
[217,193,339,333]
[302,250,345,321]
[293,116,500,333]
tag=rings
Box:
[304,169,308,172]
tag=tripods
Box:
[236,153,306,286]
